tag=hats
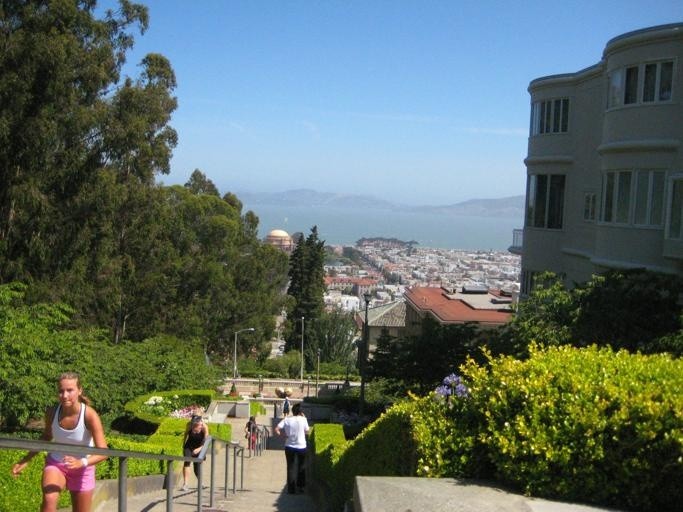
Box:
[192,409,203,416]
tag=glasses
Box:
[192,418,200,422]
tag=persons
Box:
[281,396,290,419]
[243,416,258,450]
[8,371,109,512]
[177,407,208,491]
[272,403,309,494]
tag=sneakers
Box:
[181,483,189,491]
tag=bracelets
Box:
[81,457,87,466]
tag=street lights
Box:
[299,315,305,383]
[233,328,254,379]
[362,290,371,338]
